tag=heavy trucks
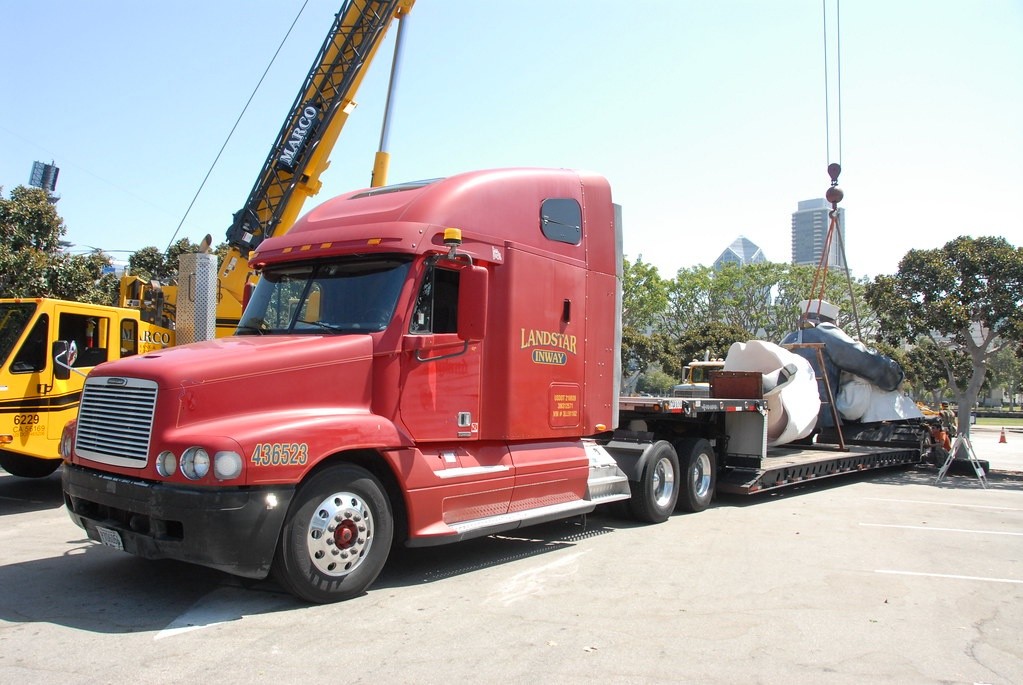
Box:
[64,171,954,605]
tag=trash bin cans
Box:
[970,411,977,424]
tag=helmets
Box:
[941,401,949,407]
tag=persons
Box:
[776,299,905,429]
[938,401,956,444]
[921,423,952,469]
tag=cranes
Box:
[1,0,414,500]
